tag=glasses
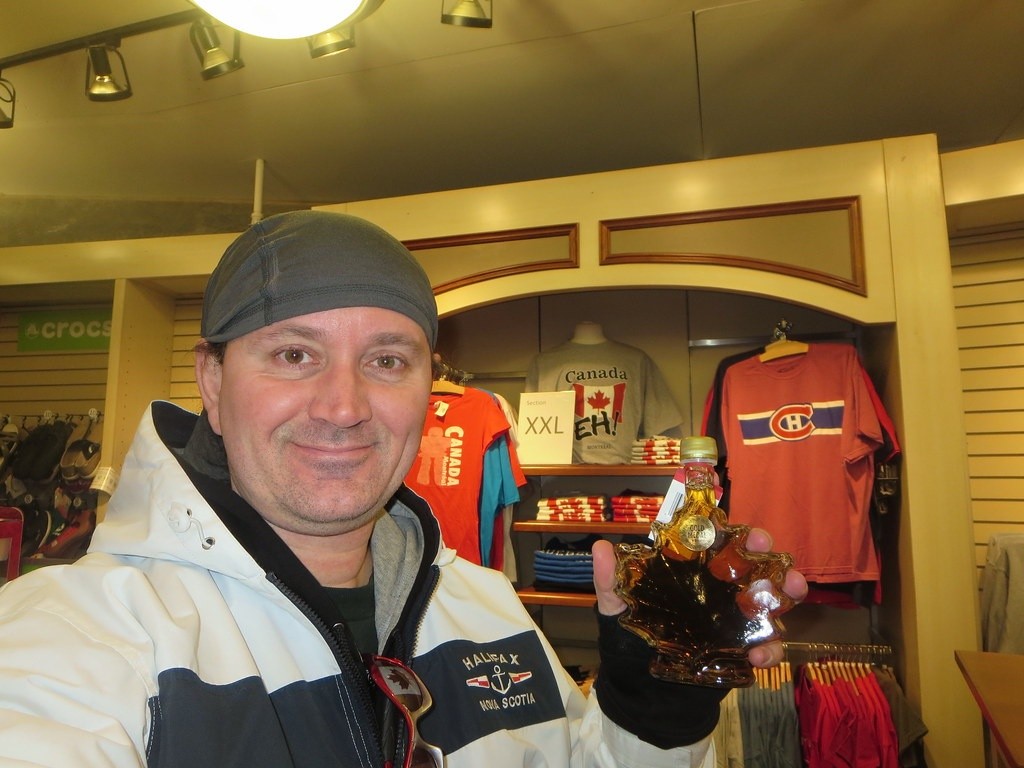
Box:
[362,652,444,768]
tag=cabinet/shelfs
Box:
[513,461,722,607]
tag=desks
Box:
[951,652,1024,768]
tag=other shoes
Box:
[1,416,105,559]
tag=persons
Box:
[0,211,810,768]
[525,320,683,464]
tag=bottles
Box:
[615,437,791,688]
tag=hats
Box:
[199,209,440,357]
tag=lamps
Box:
[1,1,499,138]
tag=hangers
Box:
[753,642,894,692]
[759,317,808,361]
[432,363,470,394]
